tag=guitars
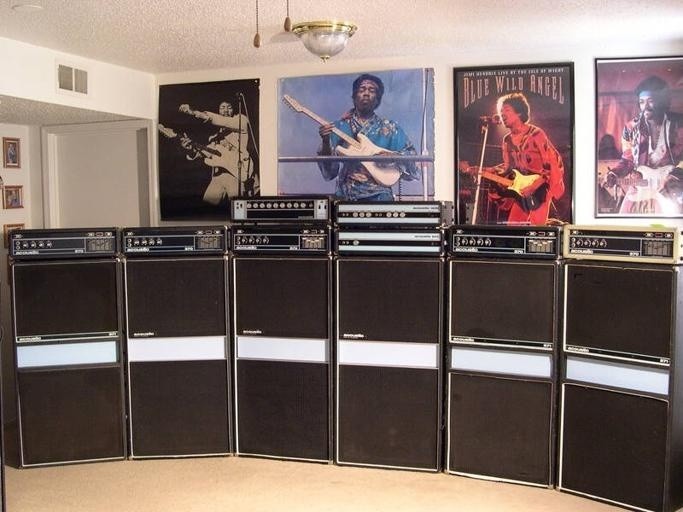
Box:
[461,162,545,211]
[597,165,679,208]
[282,94,404,186]
[156,123,254,182]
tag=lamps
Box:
[291,20,358,62]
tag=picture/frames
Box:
[0,132,24,286]
[594,54,682,219]
[451,62,575,226]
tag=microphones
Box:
[480,114,501,124]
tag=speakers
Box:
[446,258,562,489]
[230,255,333,465]
[7,257,126,470]
[557,262,683,512]
[122,256,233,460]
[335,256,446,473]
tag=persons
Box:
[315,74,419,202]
[470,93,565,225]
[177,96,254,206]
[601,76,683,214]
